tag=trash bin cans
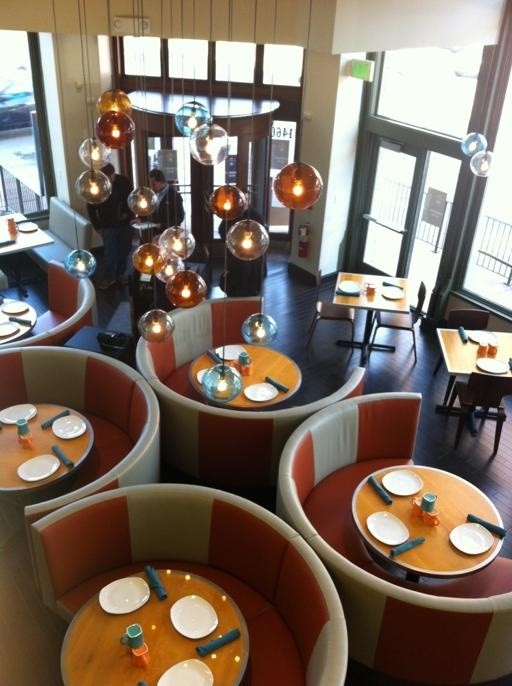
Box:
[95,331,135,363]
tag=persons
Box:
[130,168,185,290]
[86,163,135,290]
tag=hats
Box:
[98,162,115,176]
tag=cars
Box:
[0,73,37,134]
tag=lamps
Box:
[463,2,510,177]
[71,1,324,403]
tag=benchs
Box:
[3,199,512,685]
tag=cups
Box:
[14,419,35,450]
[128,643,151,669]
[8,218,17,234]
[119,624,144,649]
[408,493,441,528]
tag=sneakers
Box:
[116,274,129,285]
[97,278,116,290]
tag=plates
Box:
[465,327,511,375]
[155,659,215,686]
[198,342,278,403]
[52,415,87,439]
[448,523,494,556]
[0,321,20,337]
[336,279,406,301]
[18,222,39,232]
[98,578,150,616]
[0,404,38,425]
[2,300,30,314]
[366,510,409,548]
[18,453,62,482]
[381,470,424,498]
[170,597,221,641]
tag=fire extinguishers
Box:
[296,220,311,258]
